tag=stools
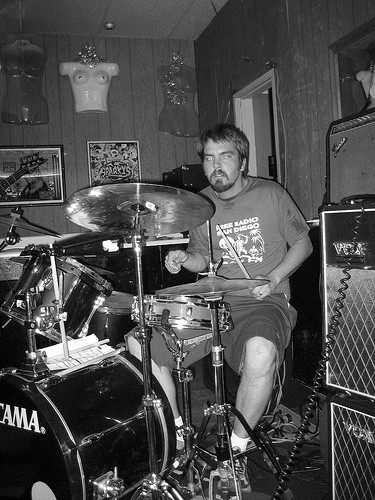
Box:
[203,332,294,408]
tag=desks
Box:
[0,232,208,368]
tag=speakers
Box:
[326,392,375,500]
[319,202,375,402]
[326,107,375,203]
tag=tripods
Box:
[69,181,215,500]
[154,256,289,500]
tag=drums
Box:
[86,290,138,348]
[0,244,115,346]
[0,349,177,500]
[131,294,234,333]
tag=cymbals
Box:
[155,276,272,296]
[0,214,61,239]
[63,183,215,240]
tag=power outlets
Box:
[273,404,319,440]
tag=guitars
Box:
[0,152,49,193]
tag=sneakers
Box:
[231,446,251,494]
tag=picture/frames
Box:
[87,140,142,188]
[0,145,66,208]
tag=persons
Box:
[124,125,313,493]
